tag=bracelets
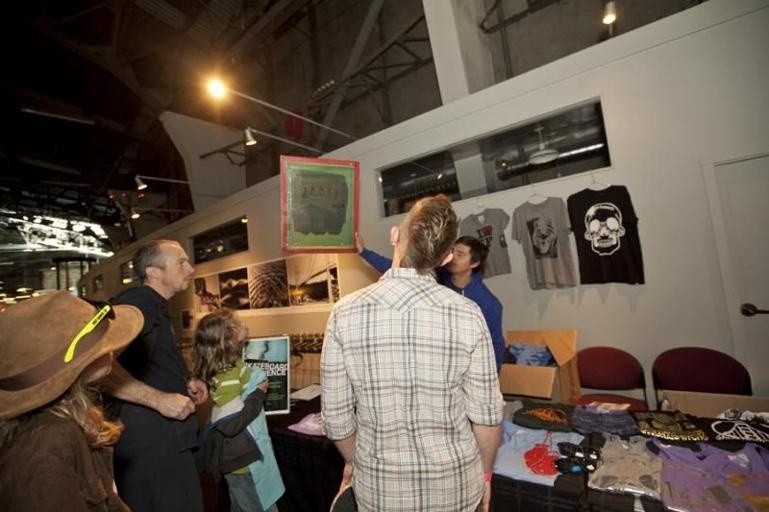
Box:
[484,472,493,481]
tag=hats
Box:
[1,290,145,419]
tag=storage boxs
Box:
[499,330,581,407]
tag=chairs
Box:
[651,347,753,410]
[576,346,648,412]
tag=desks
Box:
[265,387,769,512]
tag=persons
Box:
[0,289,145,512]
[320,194,507,512]
[99,239,210,512]
[191,309,287,512]
[356,235,503,378]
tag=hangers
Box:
[586,171,609,190]
[527,185,549,204]
[472,196,486,215]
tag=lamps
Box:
[243,127,326,156]
[135,175,189,190]
[602,1,617,39]
[530,124,558,165]
[129,207,195,219]
